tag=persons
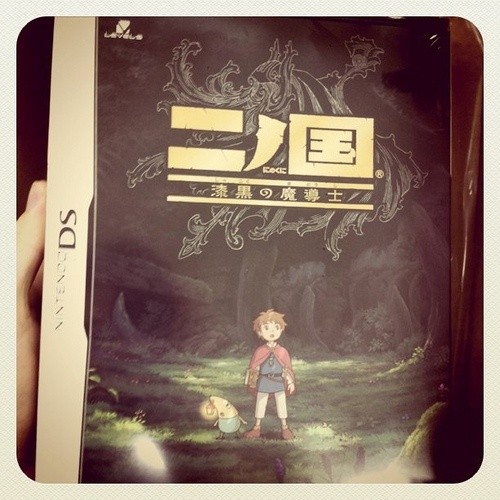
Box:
[17,181,48,480]
